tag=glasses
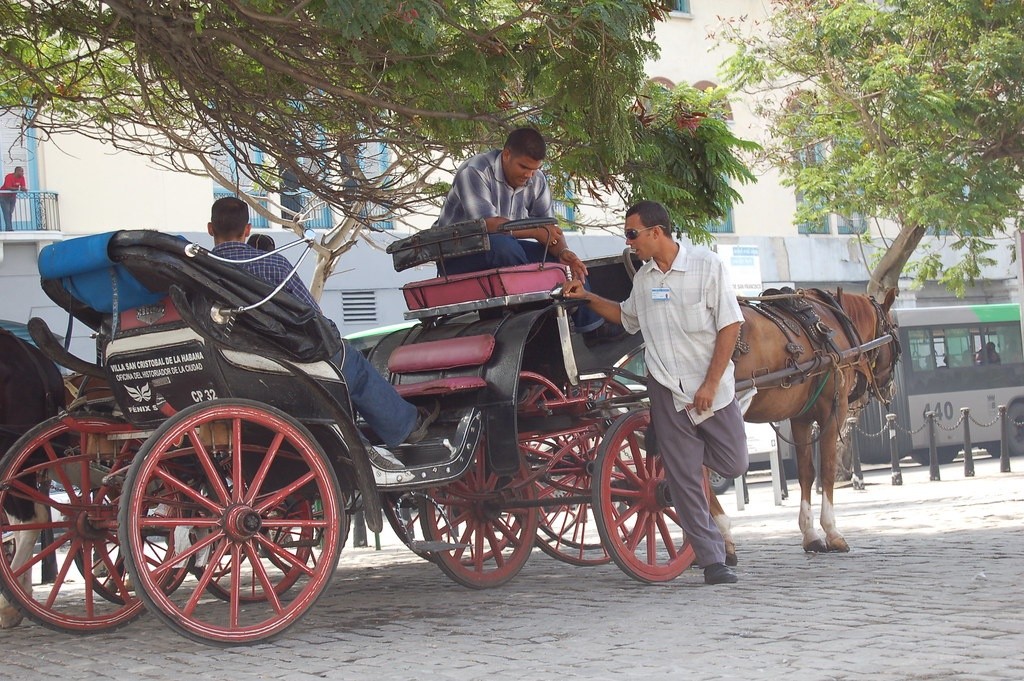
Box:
[625,225,669,240]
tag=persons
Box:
[563,201,751,584]
[431,128,629,347]
[206,196,440,447]
[986,341,1000,364]
[248,234,275,251]
[0,166,28,231]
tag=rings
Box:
[553,240,558,244]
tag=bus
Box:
[781,310,1024,479]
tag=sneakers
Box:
[645,419,662,456]
[702,563,739,585]
[406,396,441,444]
[583,324,630,347]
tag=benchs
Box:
[100,317,349,419]
[399,262,611,358]
[388,332,495,396]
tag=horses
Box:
[683,287,900,566]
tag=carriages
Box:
[0,218,905,650]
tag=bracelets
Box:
[510,230,513,235]
